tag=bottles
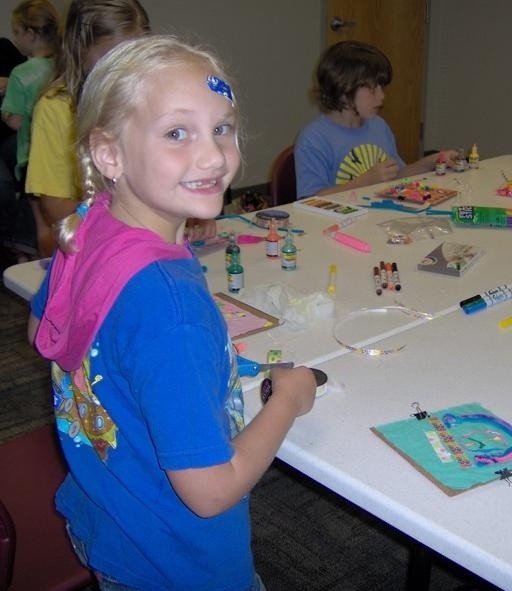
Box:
[226,217,297,294]
[434,144,479,175]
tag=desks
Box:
[0,155,512,591]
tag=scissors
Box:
[235,355,294,377]
[237,235,285,244]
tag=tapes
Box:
[309,368,328,397]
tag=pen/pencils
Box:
[300,198,358,214]
[373,261,401,295]
[460,285,512,314]
[328,264,336,295]
[332,232,370,253]
[320,218,352,235]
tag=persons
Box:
[1,0,65,260]
[24,1,153,233]
[0,34,33,295]
[27,33,319,590]
[289,41,466,201]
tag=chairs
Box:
[271,146,296,206]
[0,424,94,591]
